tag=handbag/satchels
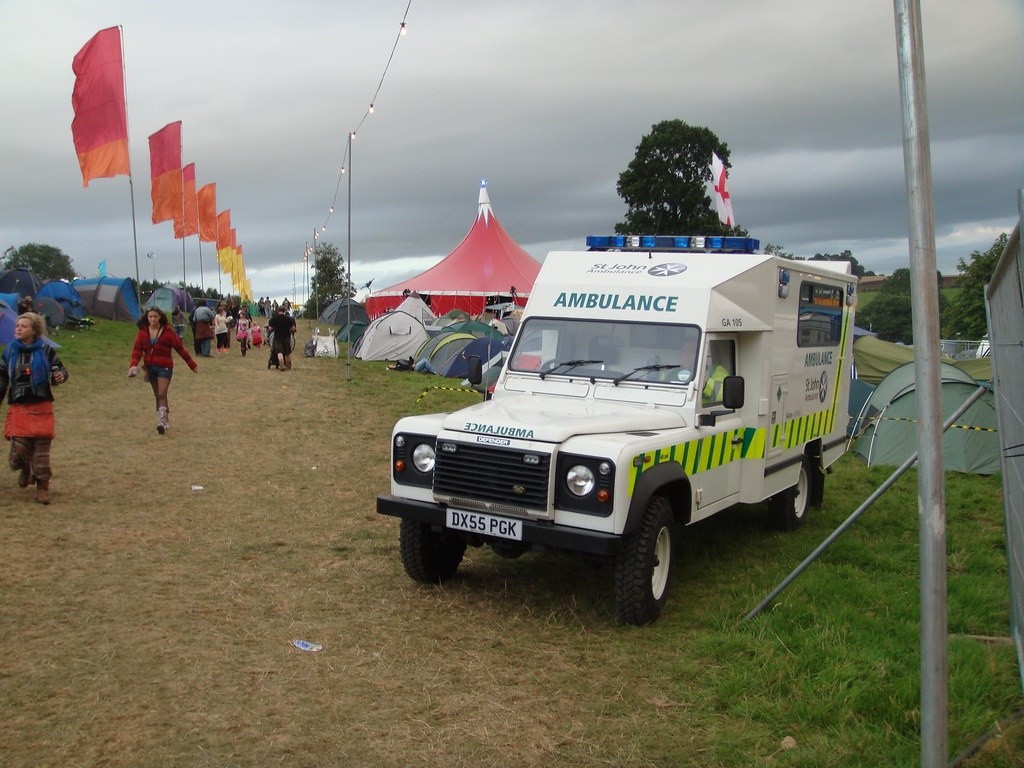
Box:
[141,366,150,382]
[236,332,240,341]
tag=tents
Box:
[0,267,85,350]
[71,277,142,324]
[320,178,545,394]
[141,282,195,318]
[846,321,1004,476]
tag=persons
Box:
[172,303,186,343]
[0,313,69,504]
[387,358,439,375]
[189,297,297,371]
[127,307,201,435]
[663,334,731,406]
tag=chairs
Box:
[574,335,625,379]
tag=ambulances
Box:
[376,234,860,625]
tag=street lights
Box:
[147,251,159,306]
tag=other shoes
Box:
[242,351,246,355]
[217,348,220,352]
[223,349,228,354]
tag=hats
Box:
[220,301,228,305]
[239,310,245,314]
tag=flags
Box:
[708,152,735,230]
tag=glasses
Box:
[148,306,158,311]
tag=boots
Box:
[18,468,31,487]
[36,479,50,502]
[157,406,169,434]
